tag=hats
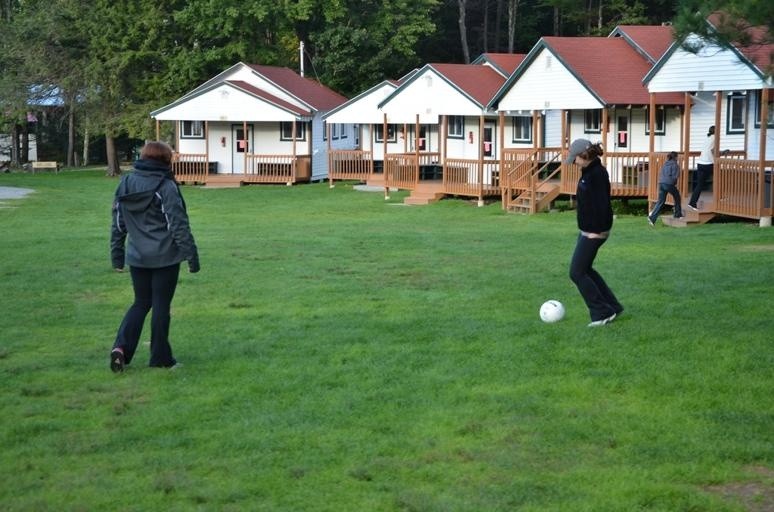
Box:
[560,138,594,165]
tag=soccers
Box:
[539,300,565,323]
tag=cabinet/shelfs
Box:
[173,162,217,174]
[257,163,292,175]
[532,162,562,180]
[394,165,468,183]
[335,160,383,174]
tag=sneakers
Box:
[587,312,618,328]
[674,215,686,219]
[687,203,700,211]
[110,347,126,375]
[647,216,655,228]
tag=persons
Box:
[647,152,685,227]
[110,140,201,373]
[561,139,625,326]
[687,126,716,212]
